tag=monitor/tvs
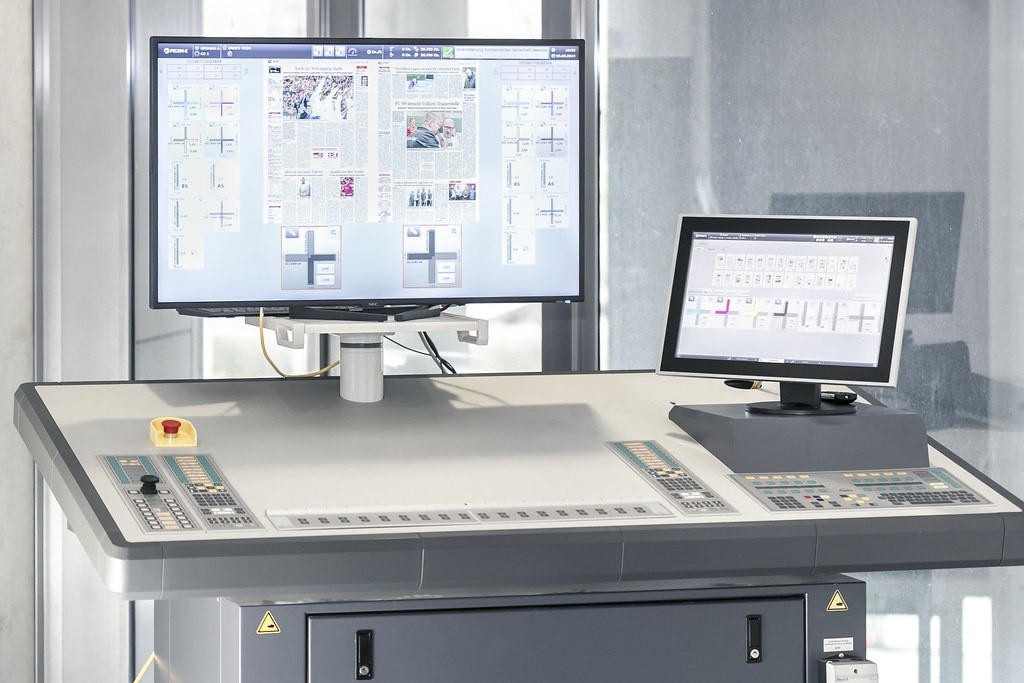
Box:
[656,212,917,418]
[149,36,585,319]
